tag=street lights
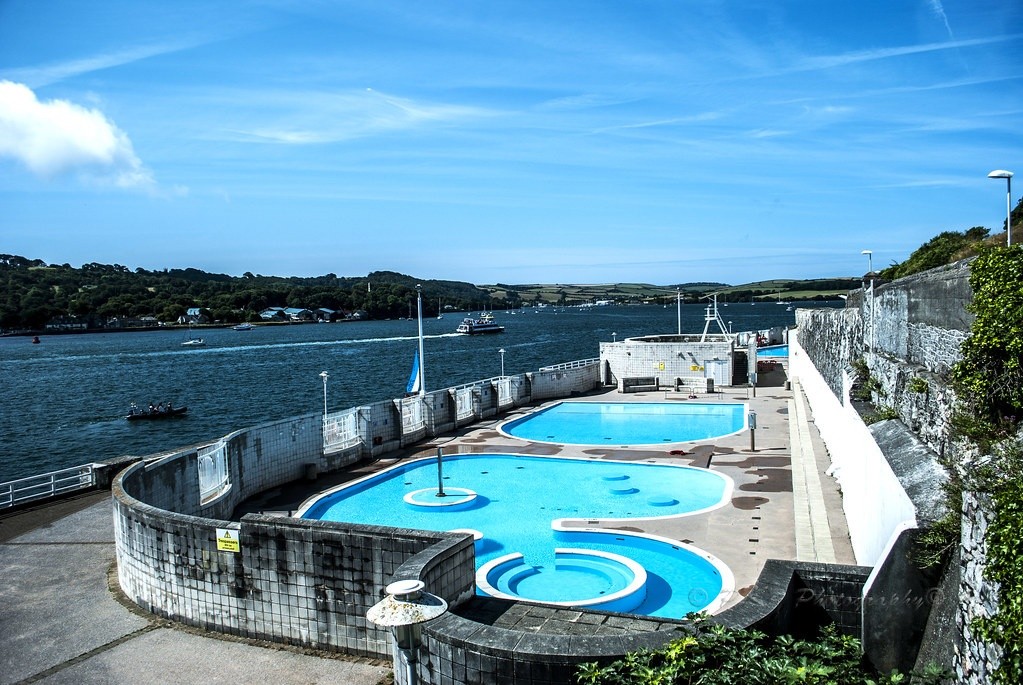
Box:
[498,348,506,376]
[365,579,450,685]
[413,284,427,394]
[611,331,618,342]
[988,168,1015,247]
[675,287,682,335]
[319,371,329,419]
[860,249,875,352]
[727,320,733,334]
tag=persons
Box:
[757,332,767,347]
[140,401,172,415]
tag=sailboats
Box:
[180,311,206,348]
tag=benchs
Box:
[618,375,660,394]
[673,376,715,394]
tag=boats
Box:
[226,322,256,331]
[498,295,795,318]
[455,307,505,336]
[123,406,188,421]
[32,336,41,344]
